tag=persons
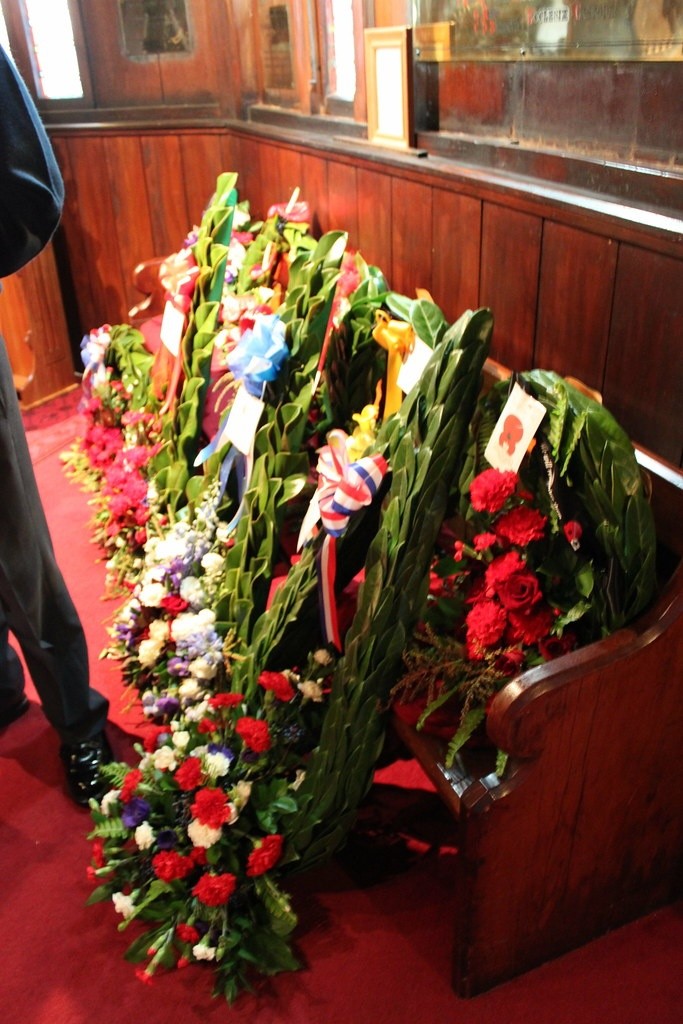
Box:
[0,44,119,808]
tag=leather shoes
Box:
[59,729,119,807]
[0,691,31,734]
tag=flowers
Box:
[85,162,653,1005]
